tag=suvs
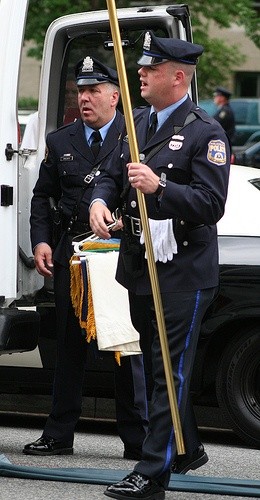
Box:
[0,1,260,444]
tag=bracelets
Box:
[153,172,166,197]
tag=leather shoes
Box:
[104,472,166,500]
[123,447,143,461]
[169,444,208,475]
[22,435,73,455]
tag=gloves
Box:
[139,217,178,263]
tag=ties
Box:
[90,130,102,160]
[146,113,157,144]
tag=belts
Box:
[122,212,204,237]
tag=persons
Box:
[212,87,236,154]
[23,58,149,460]
[89,30,231,500]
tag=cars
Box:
[197,98,260,167]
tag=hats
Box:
[137,31,204,66]
[213,87,231,99]
[75,55,120,86]
